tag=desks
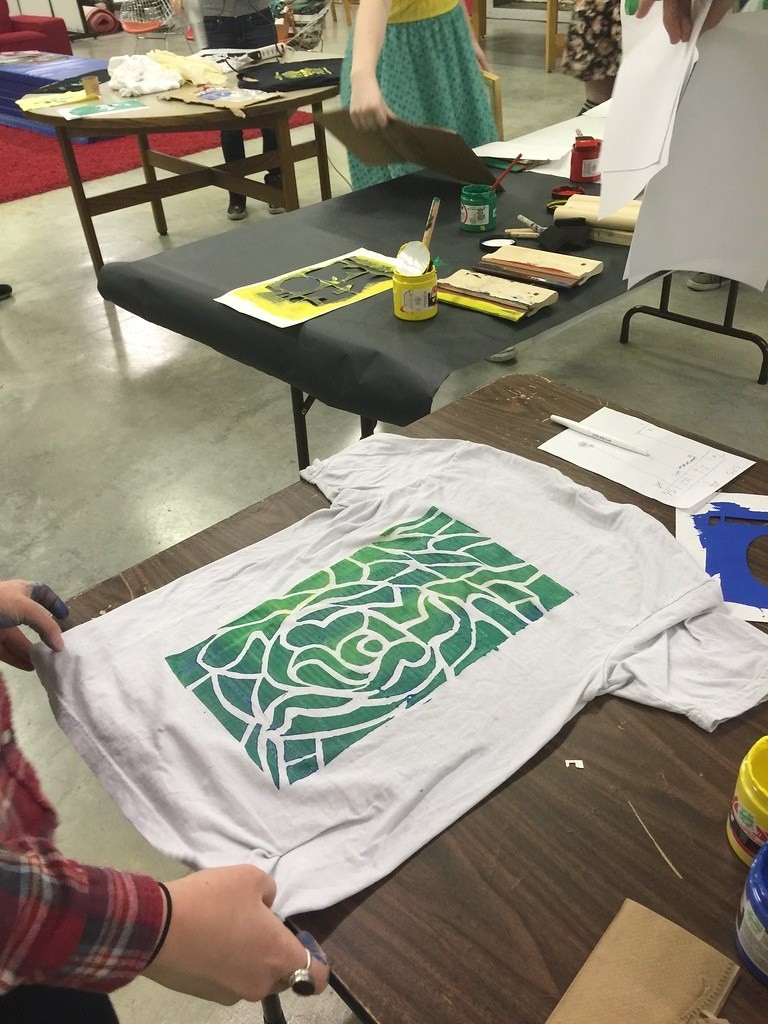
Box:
[21,52,343,279]
[95,155,768,481]
[50,371,768,1024]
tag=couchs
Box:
[0,0,73,55]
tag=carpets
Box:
[0,110,313,203]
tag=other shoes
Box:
[0,284,11,300]
[269,202,284,213]
[687,274,731,291]
[485,347,516,363]
[227,196,245,220]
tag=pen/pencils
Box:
[549,412,650,459]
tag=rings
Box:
[289,948,315,996]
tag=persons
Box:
[0,579,331,1024]
[559,0,736,292]
[171,0,285,220]
[340,0,515,363]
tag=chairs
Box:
[286,9,329,52]
[120,0,178,55]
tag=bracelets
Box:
[146,881,173,967]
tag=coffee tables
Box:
[0,52,109,145]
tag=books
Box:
[554,194,642,245]
[474,244,604,288]
[436,269,559,322]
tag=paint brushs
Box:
[504,214,548,239]
[422,197,441,247]
[490,153,523,191]
